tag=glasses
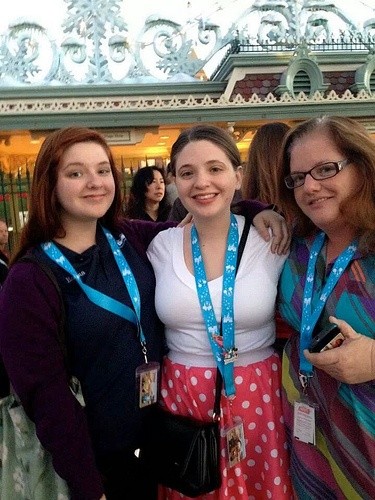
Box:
[284,158,354,189]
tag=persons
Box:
[278,115,374,499]
[0,219,12,285]
[242,122,308,230]
[126,166,175,224]
[1,127,169,500]
[146,124,298,499]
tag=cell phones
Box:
[309,323,346,353]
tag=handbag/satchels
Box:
[129,403,221,498]
[0,394,71,500]
